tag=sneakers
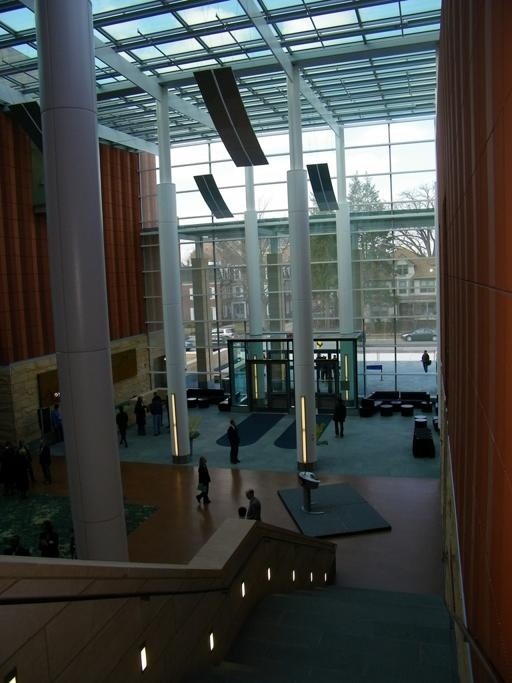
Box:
[196,495,201,503]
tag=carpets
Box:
[214,412,332,449]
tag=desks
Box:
[414,399,432,427]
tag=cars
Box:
[185,326,244,351]
[399,327,437,341]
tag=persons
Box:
[238,507,248,519]
[0,391,170,498]
[227,418,241,465]
[245,488,261,522]
[333,398,347,439]
[315,355,342,384]
[421,350,430,372]
[195,456,211,504]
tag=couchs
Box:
[359,389,429,417]
[187,388,240,412]
